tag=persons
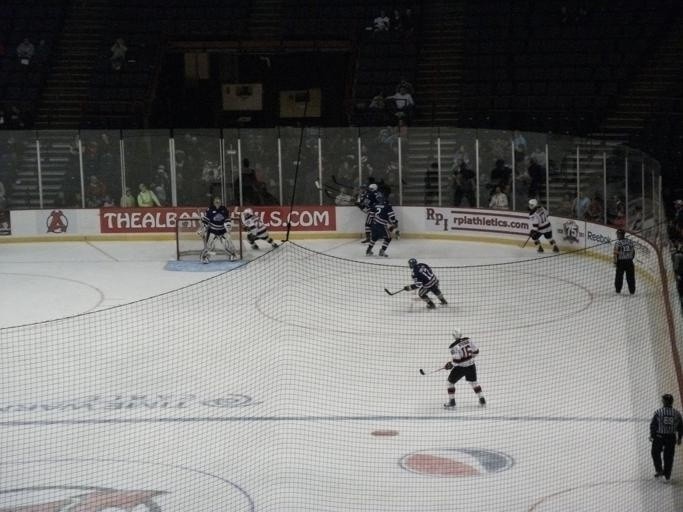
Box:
[2,104,34,178]
[358,184,400,256]
[243,124,398,205]
[571,189,643,232]
[450,129,546,213]
[56,127,221,208]
[111,39,127,69]
[401,258,449,310]
[366,75,418,128]
[645,391,683,483]
[199,196,241,263]
[613,228,636,295]
[526,198,559,254]
[17,38,50,71]
[443,329,486,406]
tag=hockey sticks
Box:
[324,188,354,203]
[385,288,405,295]
[519,238,531,247]
[420,367,445,375]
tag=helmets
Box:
[453,330,461,339]
[244,209,254,215]
[529,199,537,210]
[409,258,416,268]
[662,394,673,405]
[369,183,378,191]
